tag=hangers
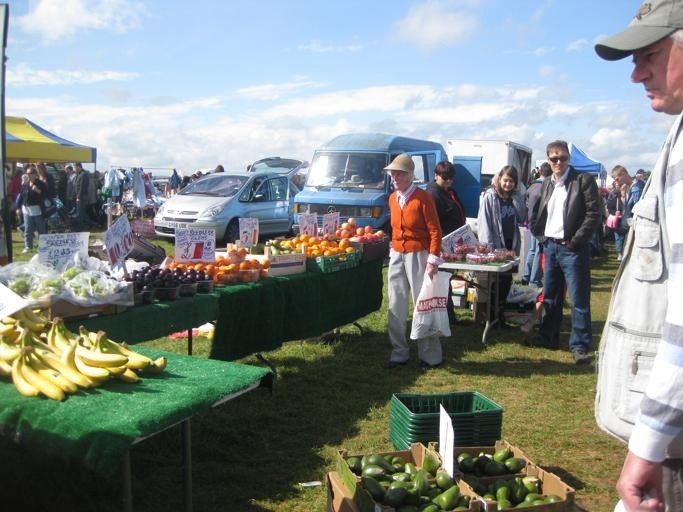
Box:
[109,166,183,235]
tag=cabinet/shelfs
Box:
[431,258,518,342]
[0,251,383,363]
[0,334,276,512]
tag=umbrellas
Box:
[2,116,96,171]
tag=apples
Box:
[320,217,384,243]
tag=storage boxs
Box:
[388,390,504,452]
[327,440,574,512]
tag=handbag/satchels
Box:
[410,270,452,340]
[606,198,620,230]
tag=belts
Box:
[549,238,564,243]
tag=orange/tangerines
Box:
[162,256,216,276]
[212,248,271,285]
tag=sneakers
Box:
[21,245,32,256]
[383,361,408,369]
[574,347,587,364]
[424,360,442,369]
[617,254,623,263]
[527,333,558,350]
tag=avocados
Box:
[345,447,562,512]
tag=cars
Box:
[155,157,312,246]
[121,179,171,214]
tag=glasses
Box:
[24,171,37,176]
[27,170,36,174]
[438,172,455,182]
[439,173,456,182]
[547,155,570,164]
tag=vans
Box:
[292,133,481,238]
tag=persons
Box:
[478,164,525,329]
[11,168,51,253]
[164,165,224,199]
[608,187,629,261]
[64,165,78,215]
[521,161,553,288]
[527,140,604,365]
[7,169,22,229]
[72,161,89,228]
[425,160,466,324]
[610,165,646,255]
[636,168,646,183]
[36,163,55,230]
[381,154,443,368]
[586,0,683,512]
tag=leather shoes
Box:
[529,281,543,289]
[522,278,527,285]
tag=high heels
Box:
[535,301,542,322]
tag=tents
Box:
[565,142,608,179]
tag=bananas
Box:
[0,316,165,400]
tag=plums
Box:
[119,266,213,301]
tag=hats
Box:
[382,152,416,174]
[595,0,683,60]
